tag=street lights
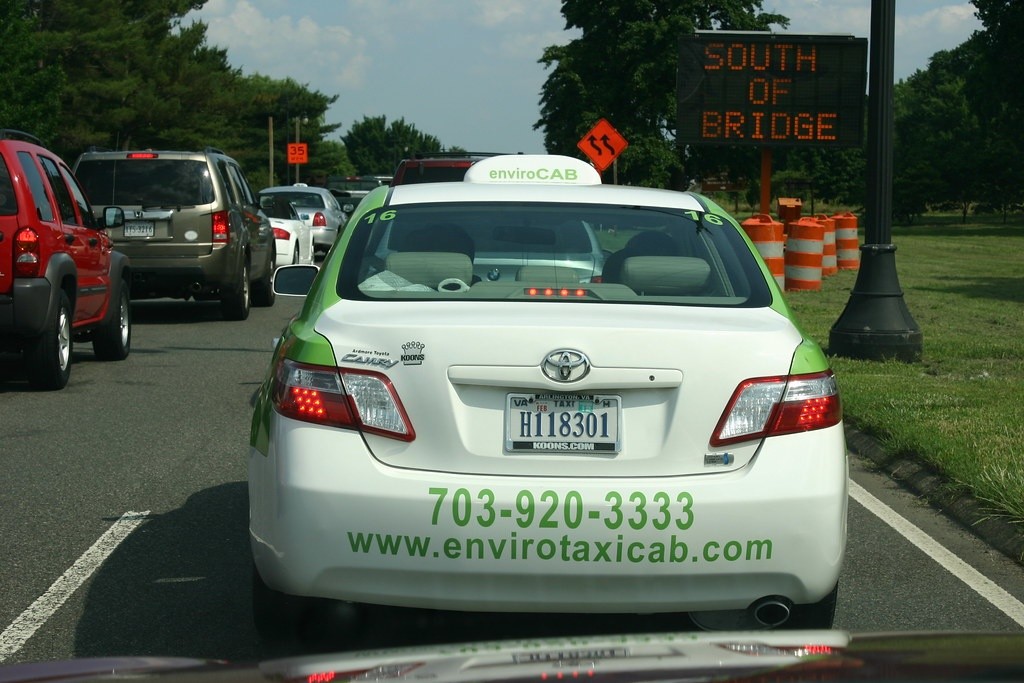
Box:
[392,136,410,174]
[285,105,310,183]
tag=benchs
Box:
[362,252,747,304]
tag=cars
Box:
[247,153,850,637]
[333,156,615,284]
[260,182,355,256]
[253,193,315,269]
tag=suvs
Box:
[0,126,135,391]
[69,145,278,322]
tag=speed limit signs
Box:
[286,143,309,165]
